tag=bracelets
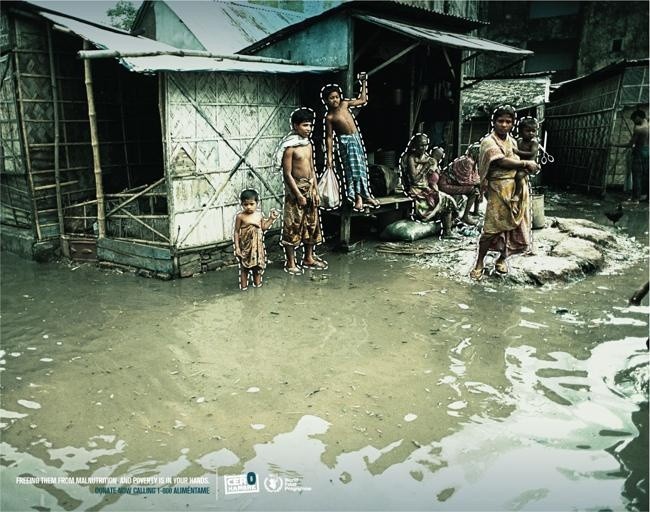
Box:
[522,160,527,168]
[424,164,428,168]
[517,150,519,154]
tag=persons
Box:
[282,110,325,273]
[438,146,483,225]
[324,72,378,209]
[610,111,649,204]
[234,190,279,288]
[470,107,542,280]
[407,135,468,238]
[631,280,649,349]
[414,149,443,210]
[510,119,538,201]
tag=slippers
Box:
[496,260,508,274]
[471,268,483,280]
[303,261,324,268]
